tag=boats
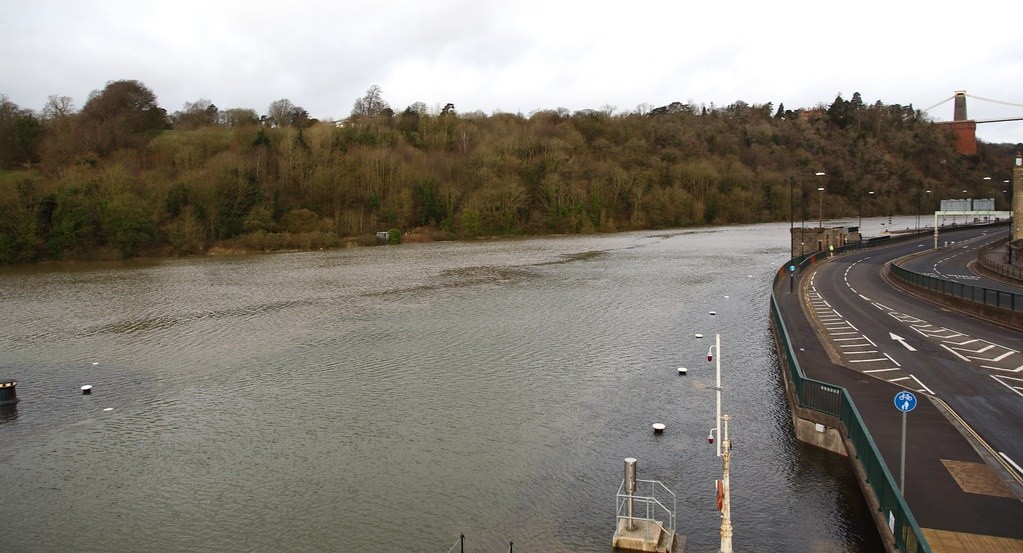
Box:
[0,376,21,406]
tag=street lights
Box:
[983,176,1012,266]
[859,192,876,230]
[918,190,931,240]
[790,172,827,293]
[818,187,825,230]
